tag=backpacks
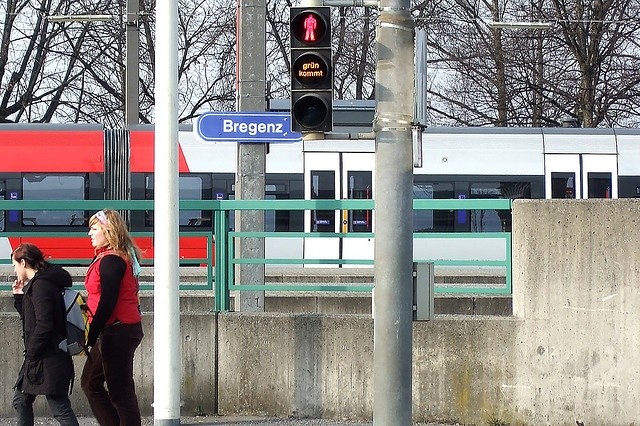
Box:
[57,287,91,357]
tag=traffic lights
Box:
[290,5,332,130]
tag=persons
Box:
[67,206,147,426]
[10,243,77,426]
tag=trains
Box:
[0,123,639,267]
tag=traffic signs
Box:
[197,111,303,142]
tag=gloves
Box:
[85,337,96,359]
